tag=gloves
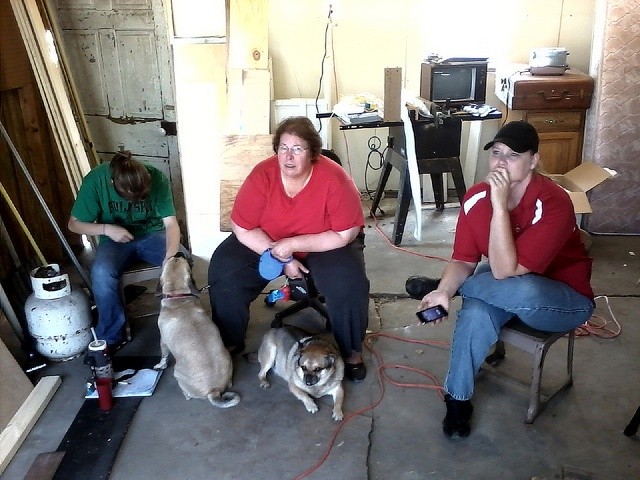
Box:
[259,247,293,280]
[288,277,310,300]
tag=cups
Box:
[96,377,114,411]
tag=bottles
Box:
[83,325,116,385]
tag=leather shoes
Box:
[345,357,367,382]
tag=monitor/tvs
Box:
[421,61,488,105]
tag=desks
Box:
[316,102,502,246]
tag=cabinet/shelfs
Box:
[501,104,583,174]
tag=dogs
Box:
[258,328,345,422]
[153,252,241,407]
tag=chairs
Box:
[267,150,372,329]
[467,227,595,425]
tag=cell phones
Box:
[415,305,449,324]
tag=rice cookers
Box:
[530,48,570,76]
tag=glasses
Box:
[276,144,311,153]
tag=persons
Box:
[68,151,193,352]
[208,116,370,383]
[405,119,596,440]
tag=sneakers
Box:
[442,393,474,441]
[405,274,460,299]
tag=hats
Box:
[483,120,539,154]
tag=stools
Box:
[121,270,175,321]
